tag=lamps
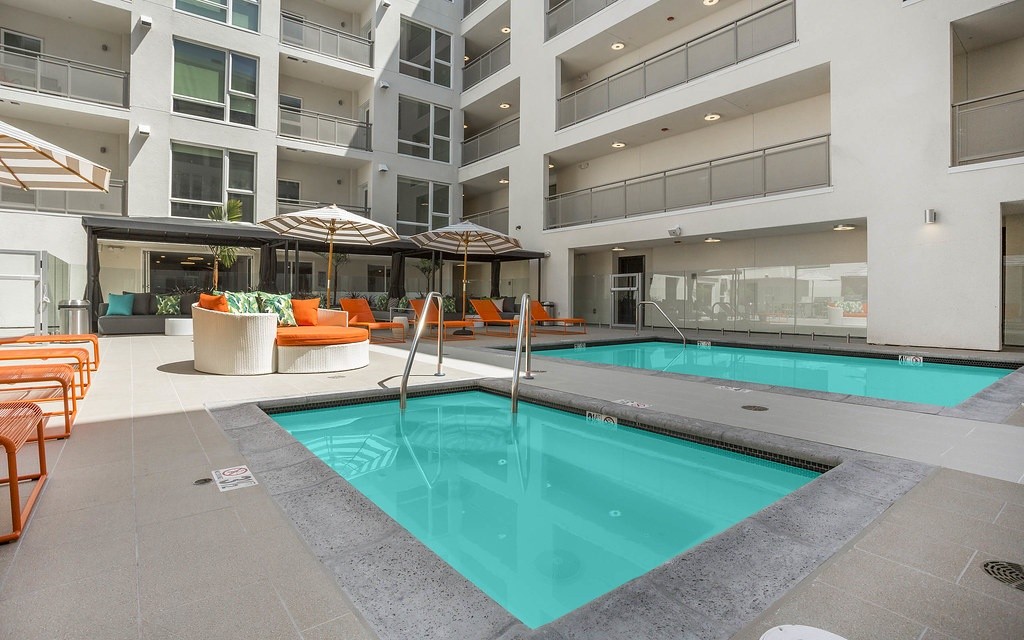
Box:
[924,209,936,224]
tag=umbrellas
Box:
[407,219,524,319]
[303,410,512,481]
[0,120,112,194]
[256,204,401,308]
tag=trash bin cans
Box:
[541,301,554,325]
[58,300,91,343]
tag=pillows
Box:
[257,291,298,327]
[123,291,151,315]
[200,290,259,313]
[398,296,409,312]
[442,298,457,313]
[492,299,505,312]
[155,293,181,316]
[106,293,134,316]
[291,296,321,326]
[180,294,196,314]
[504,297,516,313]
[375,295,387,311]
[387,297,399,312]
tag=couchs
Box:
[432,296,469,322]
[97,303,192,335]
[502,304,521,320]
[191,302,369,376]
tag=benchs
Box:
[372,297,416,332]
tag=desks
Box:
[164,318,193,335]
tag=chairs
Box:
[339,298,406,344]
[470,299,536,338]
[409,299,476,341]
[530,301,586,334]
[0,334,100,542]
